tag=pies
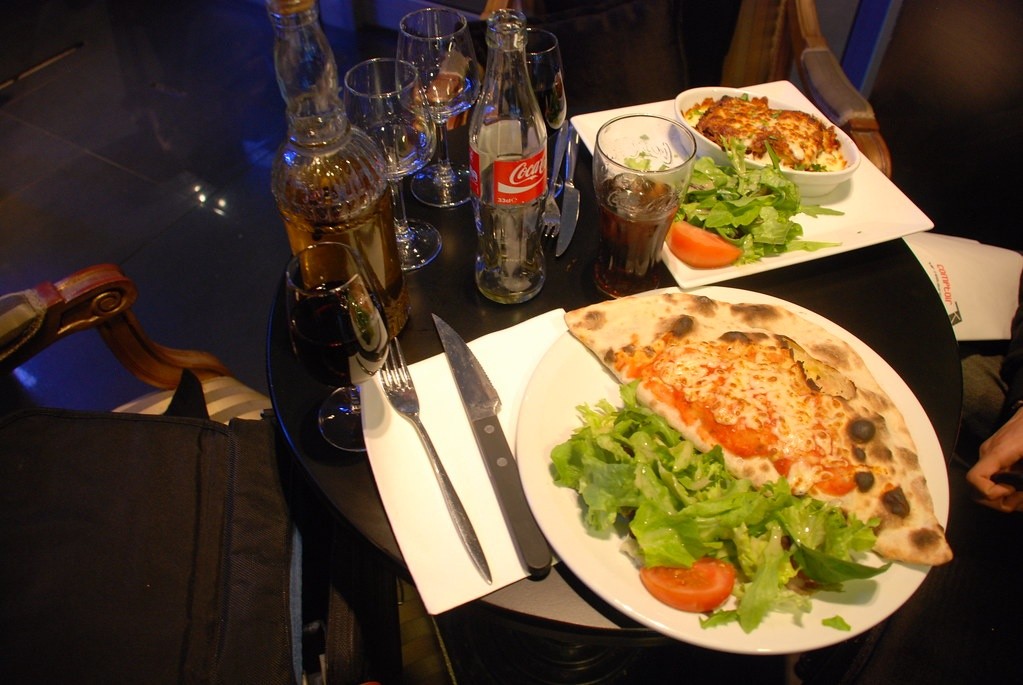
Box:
[563,292,955,566]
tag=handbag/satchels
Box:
[2,412,305,684]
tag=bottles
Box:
[467,9,548,306]
[262,1,411,341]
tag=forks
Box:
[536,121,571,241]
[379,335,495,587]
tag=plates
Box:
[510,285,950,656]
[568,80,935,290]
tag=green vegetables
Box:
[623,91,845,262]
[550,376,892,632]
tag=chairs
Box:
[481,0,893,178]
[1,264,361,685]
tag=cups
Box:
[594,114,698,300]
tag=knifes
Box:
[553,126,582,257]
[431,311,553,572]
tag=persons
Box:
[966,402,1023,513]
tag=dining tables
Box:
[265,93,1021,684]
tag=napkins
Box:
[351,310,576,614]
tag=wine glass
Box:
[521,27,566,137]
[343,56,444,272]
[396,7,482,208]
[278,239,397,452]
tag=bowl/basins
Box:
[675,85,860,199]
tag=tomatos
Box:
[642,557,737,613]
[666,220,743,268]
